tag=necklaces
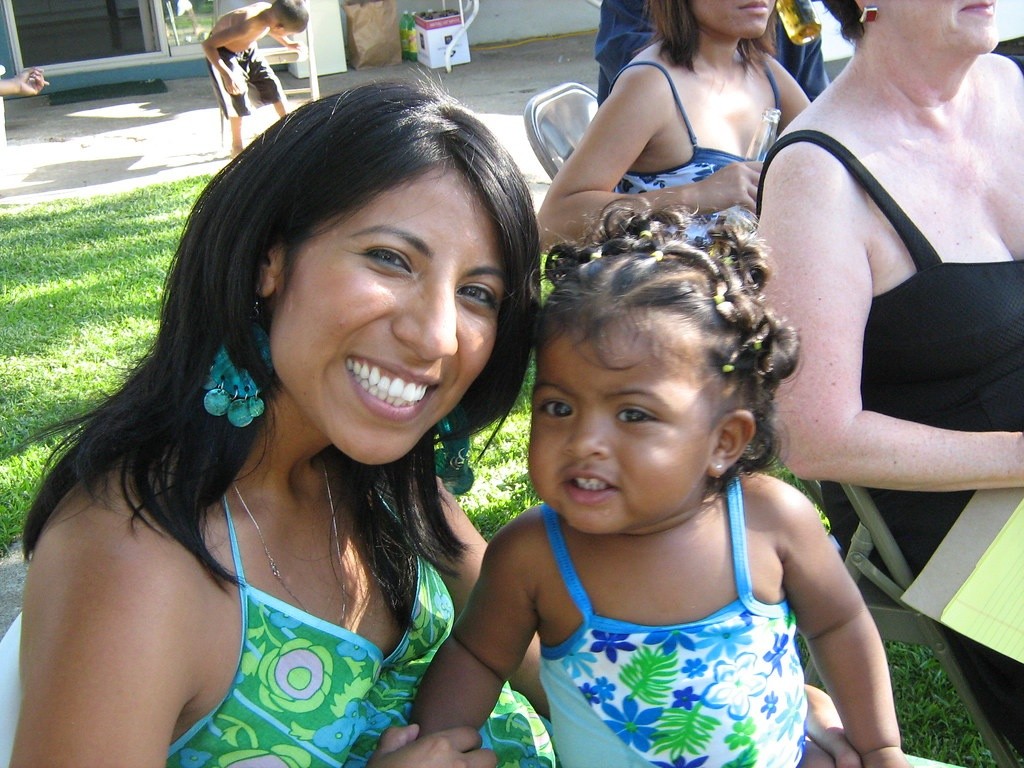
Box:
[235,469,348,626]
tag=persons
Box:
[202,0,309,159]
[408,196,913,768]
[8,81,864,768]
[756,0,1024,754]
[0,68,49,97]
[536,0,829,252]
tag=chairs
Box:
[213,0,322,148]
[525,82,599,181]
[803,481,1020,768]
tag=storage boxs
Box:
[414,9,471,68]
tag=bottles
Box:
[776,0,822,46]
[733,107,782,221]
[400,8,433,61]
